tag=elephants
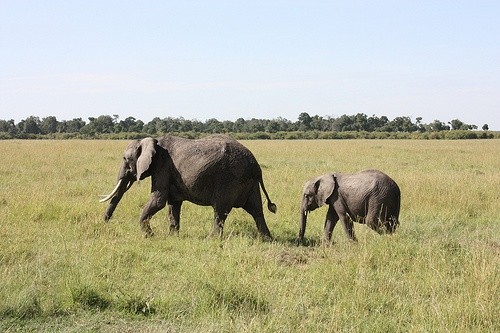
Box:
[98,134,277,243]
[296,169,401,248]
